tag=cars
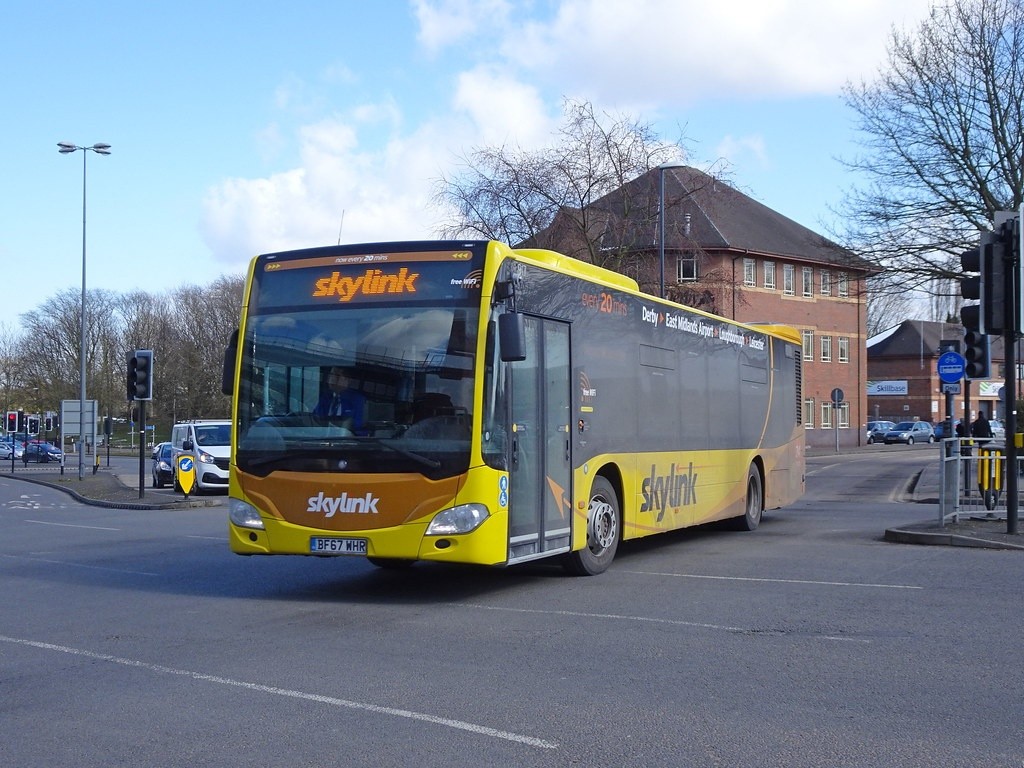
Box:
[22,443,66,463]
[866,419,896,444]
[935,419,962,439]
[883,421,935,445]
[989,419,1006,439]
[151,442,172,489]
[0,432,47,460]
[22,440,61,451]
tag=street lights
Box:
[960,229,1002,335]
[654,162,687,300]
[55,140,112,479]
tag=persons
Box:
[971,411,993,444]
[313,368,369,437]
[955,418,964,435]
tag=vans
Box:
[171,419,241,494]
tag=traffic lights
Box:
[28,416,36,435]
[45,418,51,431]
[961,333,991,383]
[34,419,40,435]
[131,349,153,402]
[6,411,18,433]
[17,411,26,432]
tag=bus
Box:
[220,239,810,575]
